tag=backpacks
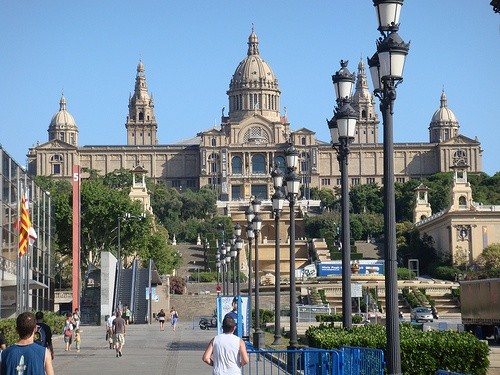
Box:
[34,323,45,343]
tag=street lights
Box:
[283,142,301,374]
[117,212,147,261]
[216,193,265,350]
[367,0,412,375]
[270,161,286,346]
[325,58,359,328]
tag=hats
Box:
[233,297,243,304]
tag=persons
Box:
[171,309,178,330]
[0,333,5,352]
[202,317,249,375]
[495,326,498,342]
[223,297,244,335]
[216,282,222,295]
[115,301,130,325]
[0,312,55,375]
[157,309,166,331]
[33,312,54,360]
[476,326,482,340]
[74,330,80,352]
[106,312,116,349]
[64,315,75,351]
[73,308,80,330]
[170,306,175,313]
[112,312,126,357]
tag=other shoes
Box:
[119,351,122,356]
[116,354,118,357]
[65,349,68,351]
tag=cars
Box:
[410,307,434,322]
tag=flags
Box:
[19,195,30,256]
[25,199,37,246]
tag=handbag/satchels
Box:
[175,312,178,318]
[66,328,71,336]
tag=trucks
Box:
[459,278,500,335]
